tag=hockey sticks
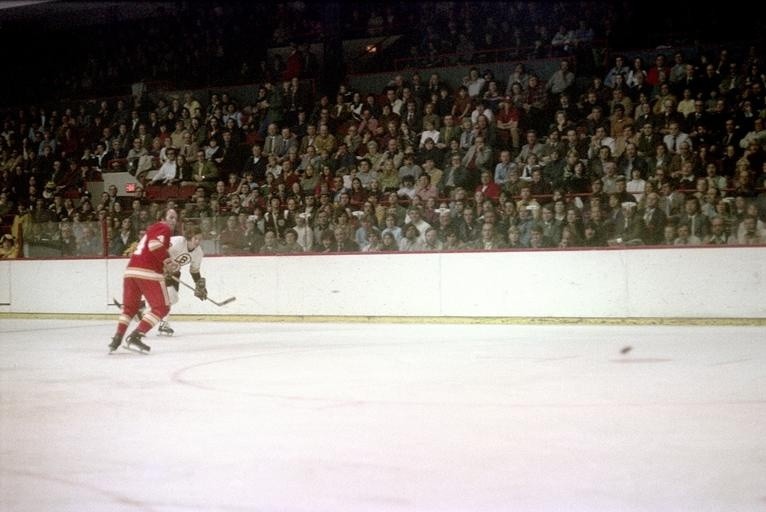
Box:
[170,275,236,307]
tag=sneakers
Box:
[159,320,174,332]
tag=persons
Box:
[132,226,208,335]
[0,1,766,263]
[107,206,179,352]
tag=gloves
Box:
[194,278,207,301]
[162,257,180,275]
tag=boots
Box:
[109,333,123,351]
[126,329,151,350]
